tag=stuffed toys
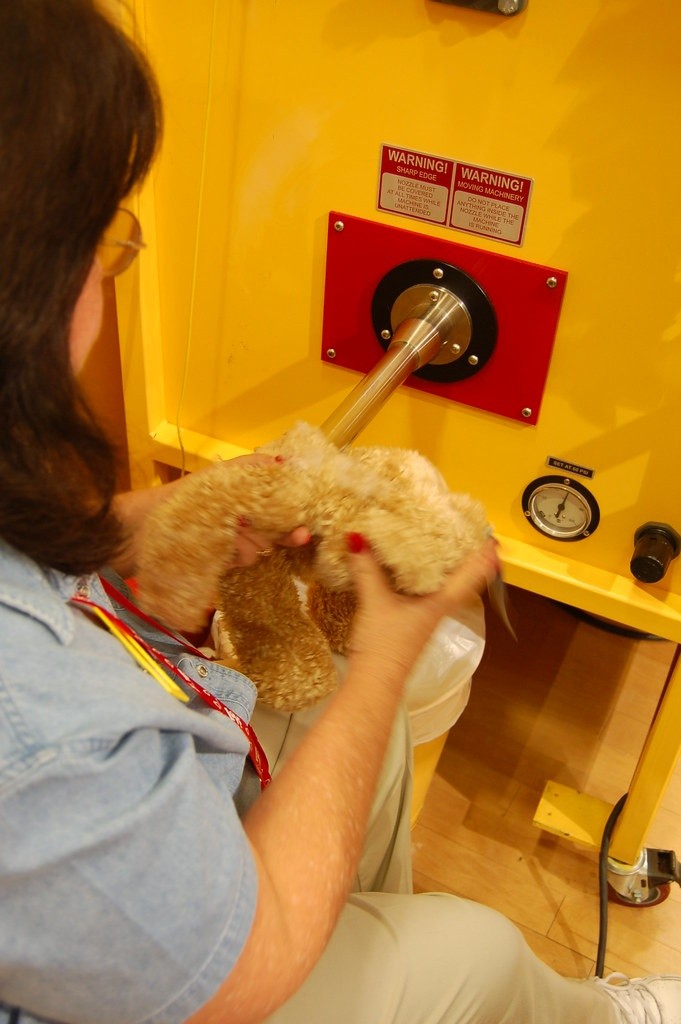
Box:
[135,423,491,711]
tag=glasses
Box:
[95,207,144,276]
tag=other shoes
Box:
[593,972,681,1024]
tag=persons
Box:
[0,0,681,1024]
